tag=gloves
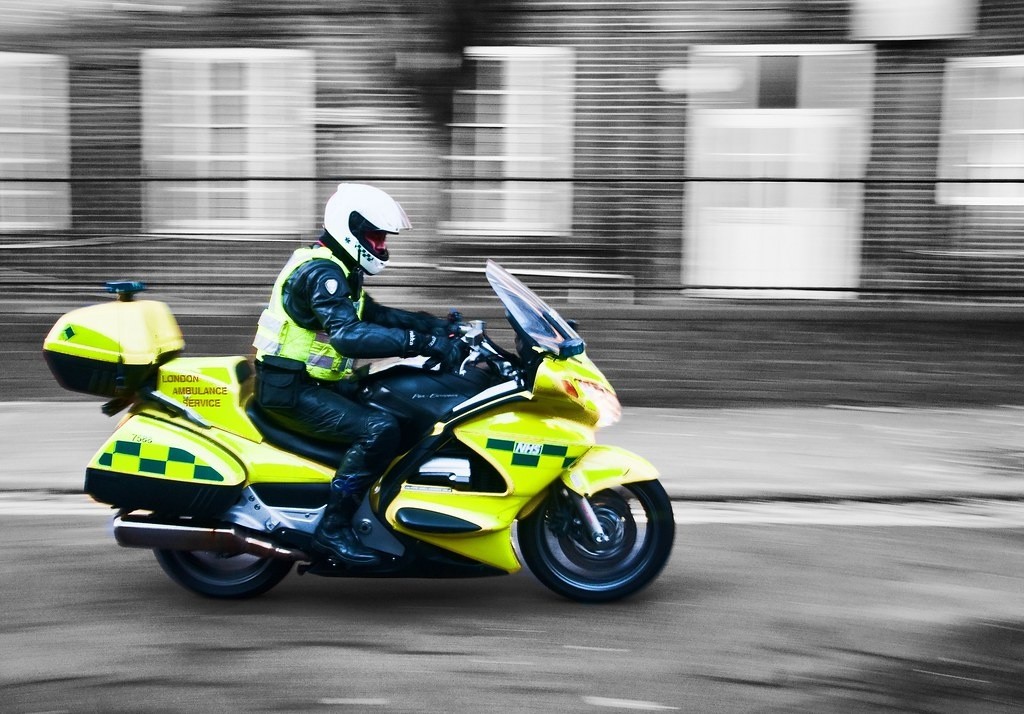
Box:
[416,311,467,337]
[405,329,461,364]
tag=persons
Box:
[254,182,458,563]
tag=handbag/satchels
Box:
[258,354,307,409]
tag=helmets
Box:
[323,182,402,277]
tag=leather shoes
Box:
[311,526,383,565]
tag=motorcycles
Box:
[40,257,677,605]
